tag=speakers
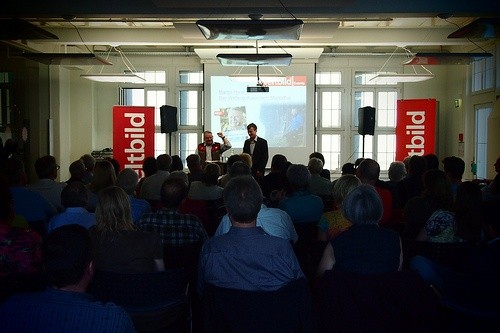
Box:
[160,105,178,133]
[358,107,375,136]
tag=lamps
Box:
[402,12,500,66]
[195,0,304,81]
[369,46,435,82]
[0,0,147,83]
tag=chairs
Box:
[0,197,500,333]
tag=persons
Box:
[227,109,246,130]
[198,131,231,163]
[243,123,268,177]
[285,109,304,145]
[0,153,500,333]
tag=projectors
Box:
[247,87,269,92]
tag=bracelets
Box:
[222,136,225,140]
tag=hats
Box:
[308,157,323,170]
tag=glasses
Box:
[56,165,60,170]
